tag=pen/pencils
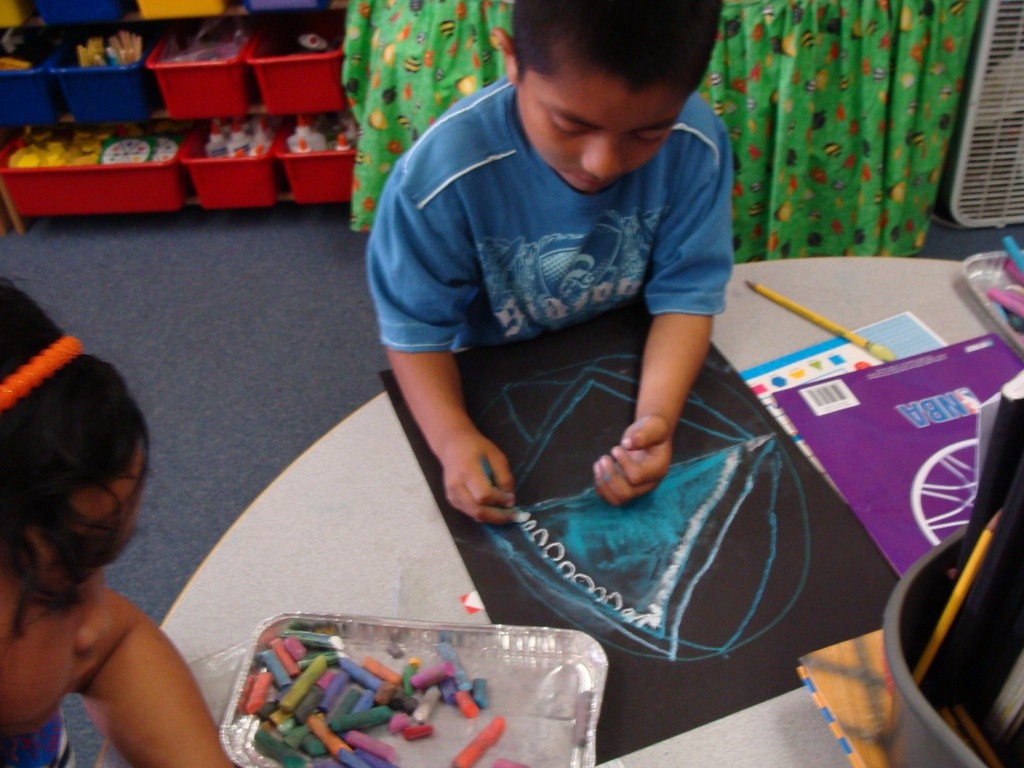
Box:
[910,507,1006,684]
[745,279,897,364]
[940,704,1001,768]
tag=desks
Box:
[93,257,1024,768]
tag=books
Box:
[773,331,1024,584]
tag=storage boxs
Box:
[0,0,356,216]
[882,529,991,768]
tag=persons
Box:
[0,270,238,768]
[365,1,734,524]
